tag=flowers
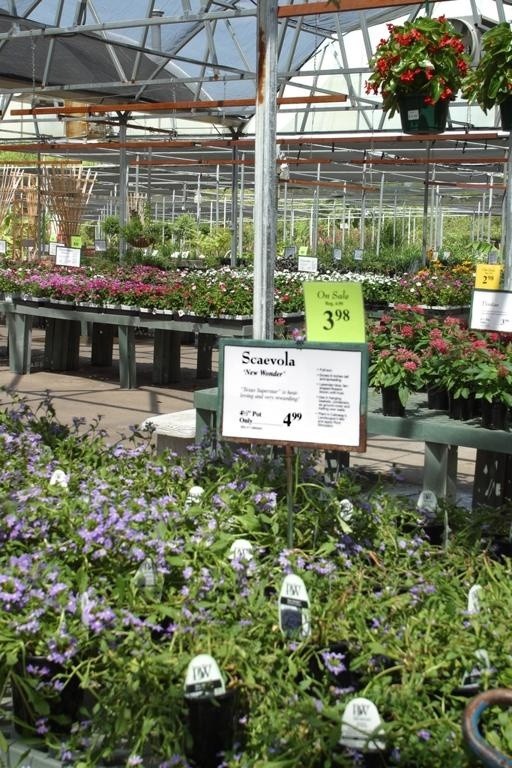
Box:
[362,9,468,119]
[271,262,510,405]
[0,264,254,319]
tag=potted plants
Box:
[471,20,512,133]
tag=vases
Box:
[381,387,507,430]
[396,95,449,135]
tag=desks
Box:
[1,301,255,391]
[191,383,512,511]
